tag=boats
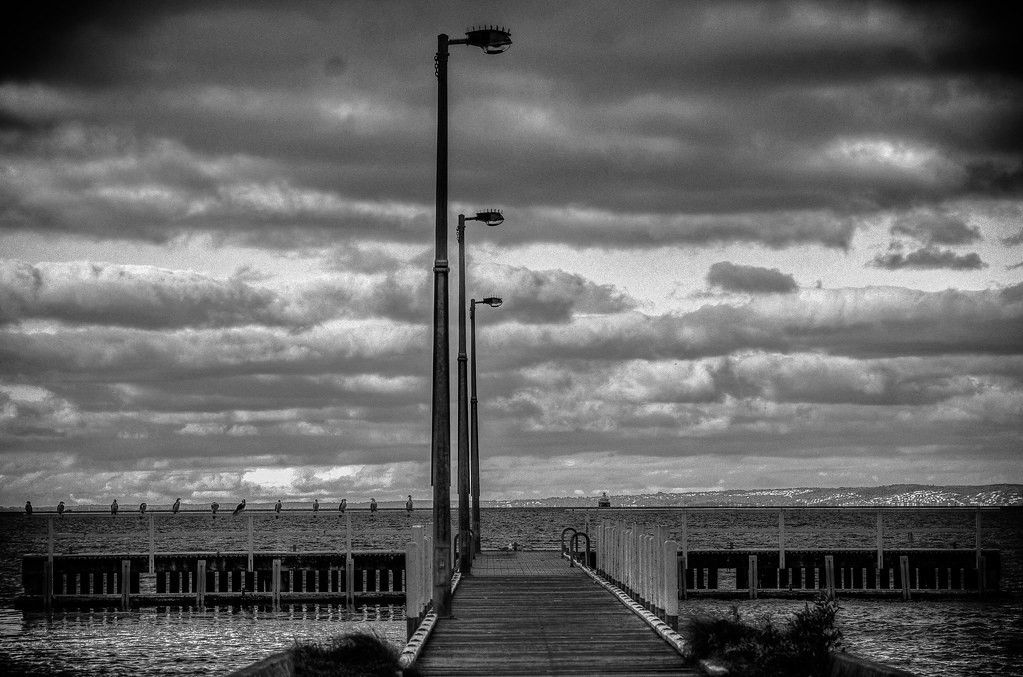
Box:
[598,492,610,507]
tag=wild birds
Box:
[232,499,246,515]
[405,495,414,518]
[24,501,32,516]
[138,503,147,520]
[274,499,282,519]
[338,499,347,518]
[110,499,118,519]
[172,498,181,514]
[312,499,319,519]
[369,498,378,523]
[211,502,219,519]
[57,502,65,515]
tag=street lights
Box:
[469,296,503,553]
[431,24,513,617]
[456,208,505,574]
